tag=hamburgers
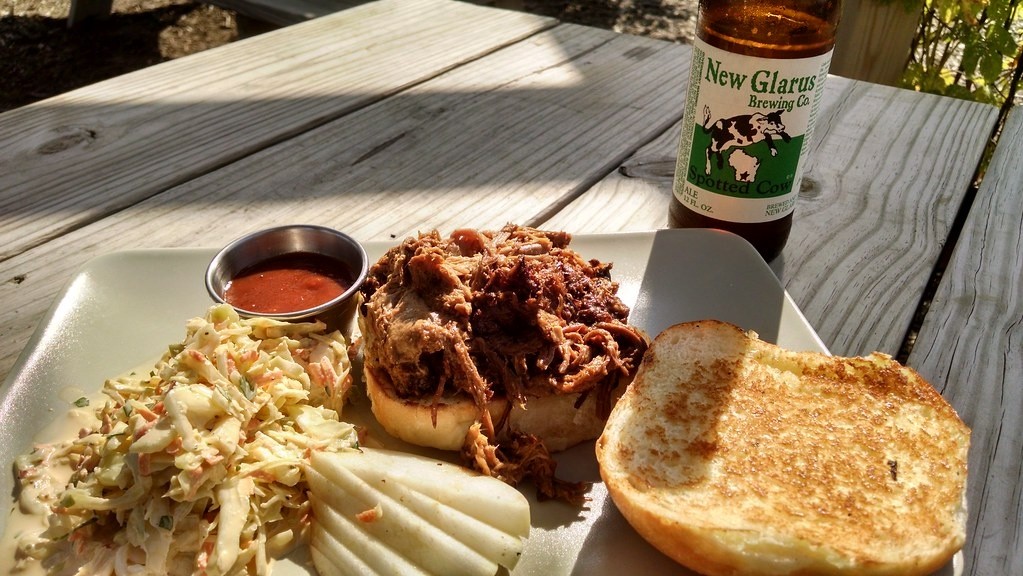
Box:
[353,219,973,575]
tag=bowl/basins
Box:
[204,223,370,340]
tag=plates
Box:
[5,230,966,576]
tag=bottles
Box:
[663,0,843,264]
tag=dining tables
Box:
[0,0,1022,576]
